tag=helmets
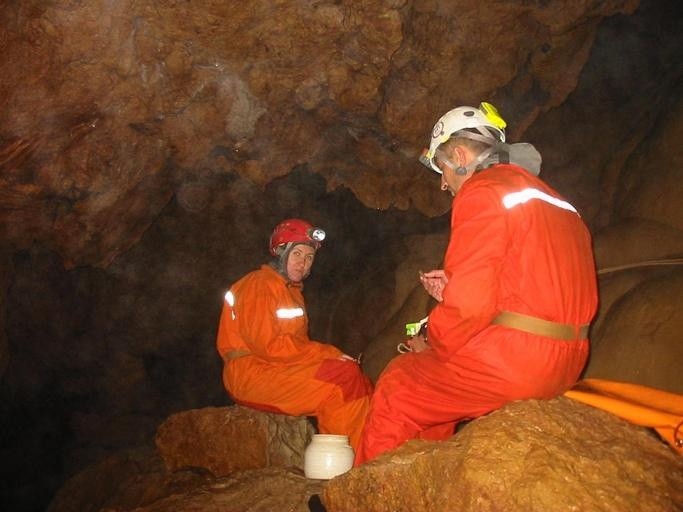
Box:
[428,106,507,174]
[270,218,320,256]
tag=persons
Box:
[308,103,599,512]
[217,218,374,454]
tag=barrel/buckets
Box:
[304,433,353,479]
